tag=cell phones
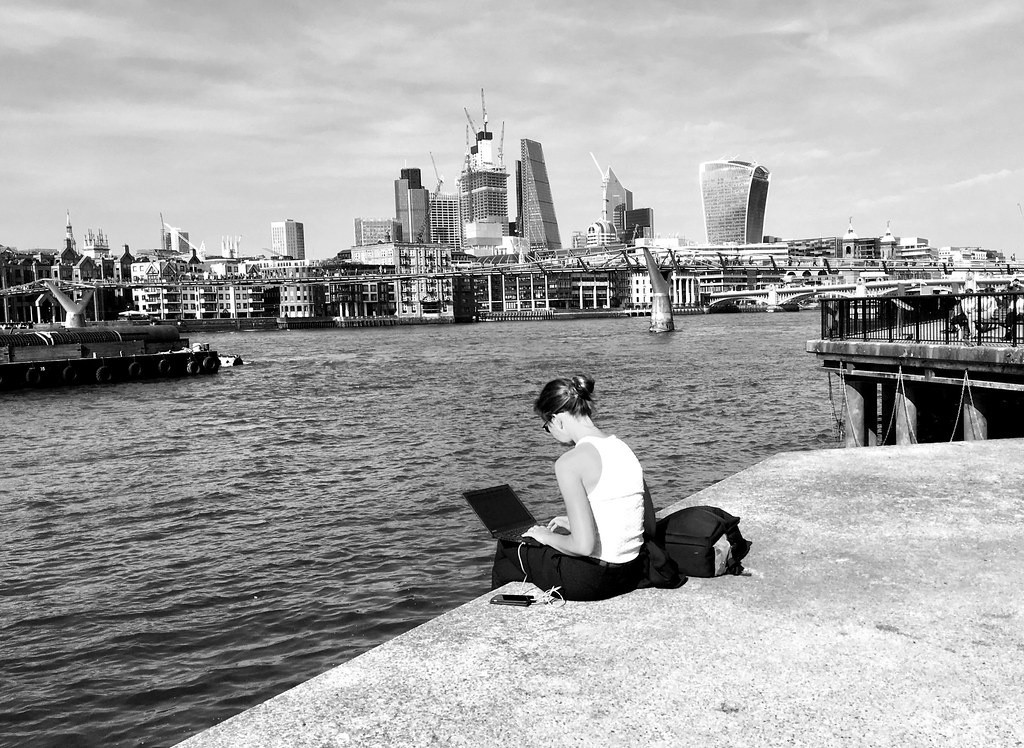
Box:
[490,594,531,603]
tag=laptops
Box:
[461,484,571,547]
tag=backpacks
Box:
[656,506,750,576]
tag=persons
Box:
[490,375,644,602]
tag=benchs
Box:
[962,307,1024,324]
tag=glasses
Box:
[541,398,570,432]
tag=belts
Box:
[588,554,643,568]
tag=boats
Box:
[219,354,243,367]
[0,324,221,393]
